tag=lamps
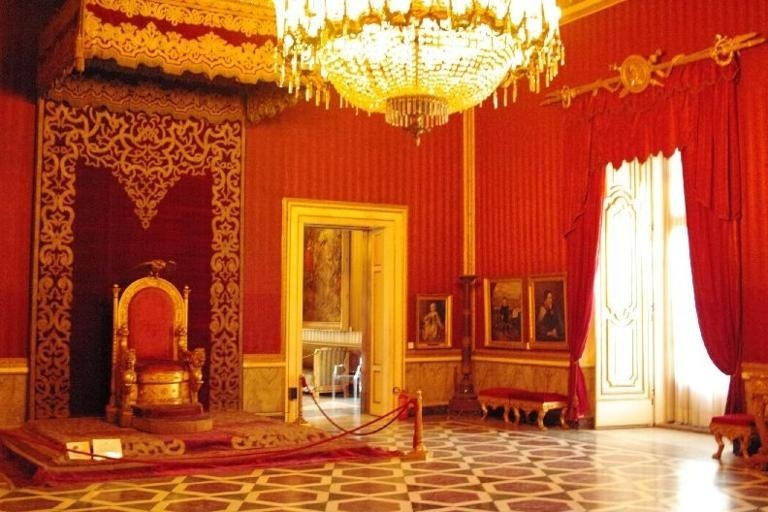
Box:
[275,1,567,147]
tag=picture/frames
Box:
[304,226,351,332]
[415,291,453,350]
[483,273,568,353]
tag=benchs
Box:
[476,386,569,430]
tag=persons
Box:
[423,302,444,341]
[500,296,509,335]
[536,288,563,341]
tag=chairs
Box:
[104,259,214,434]
[709,361,768,459]
[303,347,362,400]
